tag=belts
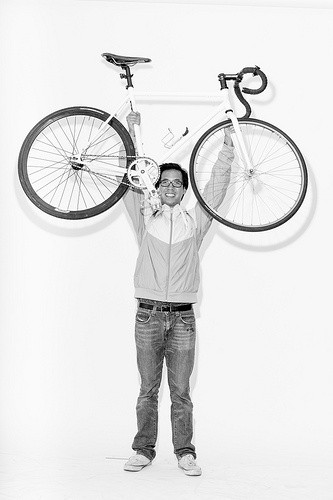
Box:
[138,302,193,312]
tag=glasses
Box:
[160,180,183,188]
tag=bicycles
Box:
[18,53,308,231]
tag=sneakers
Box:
[123,451,152,472]
[178,455,202,475]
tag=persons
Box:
[117,111,234,476]
